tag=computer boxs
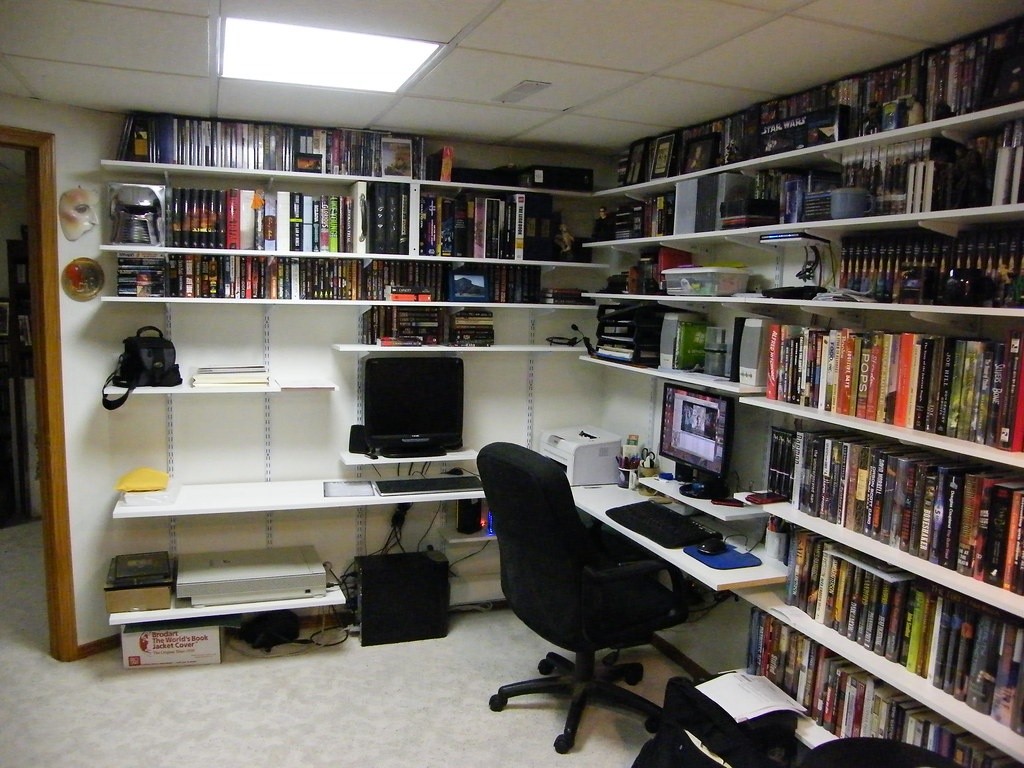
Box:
[353,551,449,646]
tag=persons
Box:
[591,205,615,242]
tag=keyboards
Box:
[373,475,484,496]
[605,500,723,550]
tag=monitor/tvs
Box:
[364,357,464,458]
[659,382,735,483]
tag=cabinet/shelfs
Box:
[661,266,750,296]
[99,99,1024,768]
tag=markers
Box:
[616,456,640,481]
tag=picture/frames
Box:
[0,298,13,338]
[680,132,721,176]
[649,135,676,181]
[450,271,490,303]
[623,136,651,186]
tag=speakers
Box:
[349,425,370,454]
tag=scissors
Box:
[642,448,655,460]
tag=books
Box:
[746,527,1024,768]
[115,113,426,181]
[628,246,692,295]
[767,426,1024,596]
[766,325,1024,454]
[840,220,1024,308]
[643,117,1024,238]
[115,251,596,305]
[618,16,1024,187]
[165,179,524,262]
[361,306,495,347]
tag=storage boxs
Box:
[122,622,229,667]
[526,164,595,194]
[104,585,173,613]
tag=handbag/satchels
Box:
[102,325,182,411]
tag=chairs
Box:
[476,441,691,755]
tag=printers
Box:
[539,424,623,486]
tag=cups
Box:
[830,188,873,219]
[764,527,786,559]
[637,466,659,496]
[617,467,638,490]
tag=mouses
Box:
[697,537,726,555]
[447,467,464,475]
